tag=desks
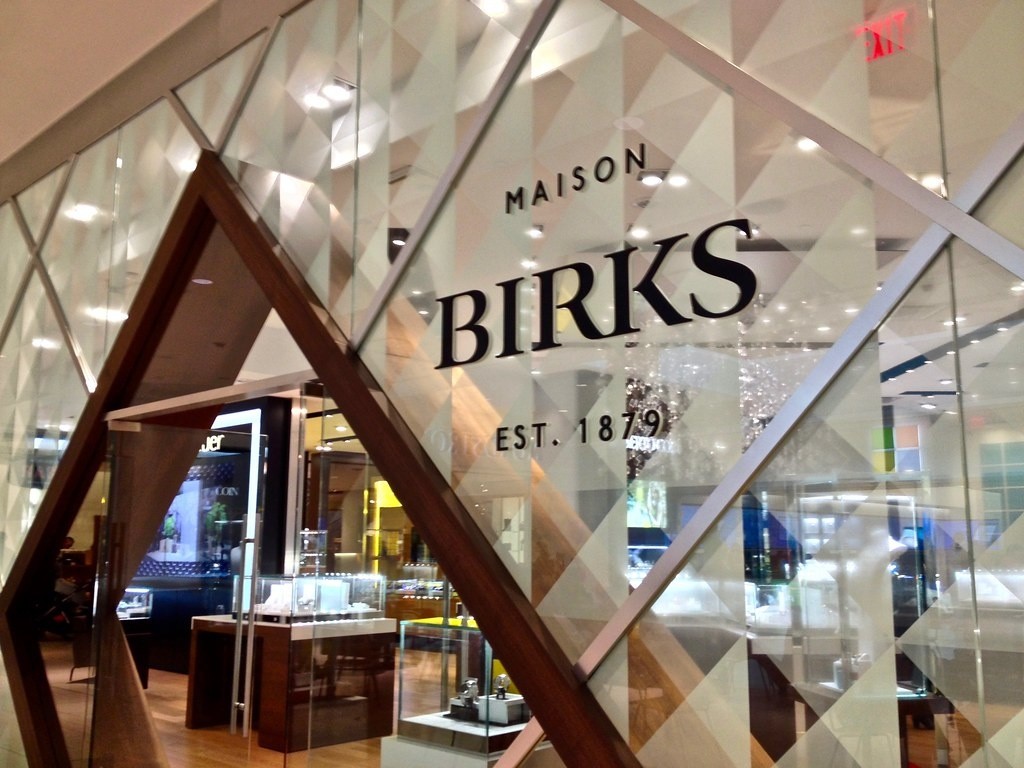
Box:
[183,615,398,755]
